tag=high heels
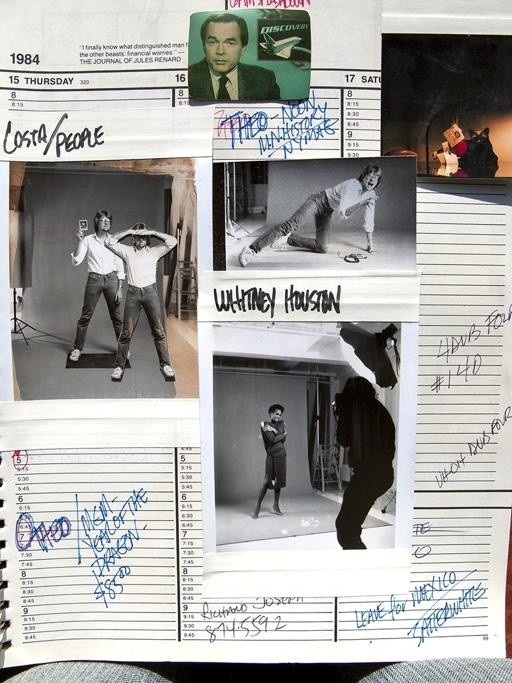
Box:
[272,504,283,515]
[252,513,258,519]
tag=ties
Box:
[217,75,230,101]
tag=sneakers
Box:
[270,233,291,248]
[160,366,175,378]
[239,246,256,267]
[111,366,124,380]
[70,350,81,362]
[126,351,131,360]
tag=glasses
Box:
[97,218,111,223]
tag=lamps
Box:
[338,323,397,388]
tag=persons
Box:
[250,404,288,519]
[188,13,281,103]
[328,375,396,549]
[70,208,131,361]
[235,162,382,266]
[104,221,178,382]
